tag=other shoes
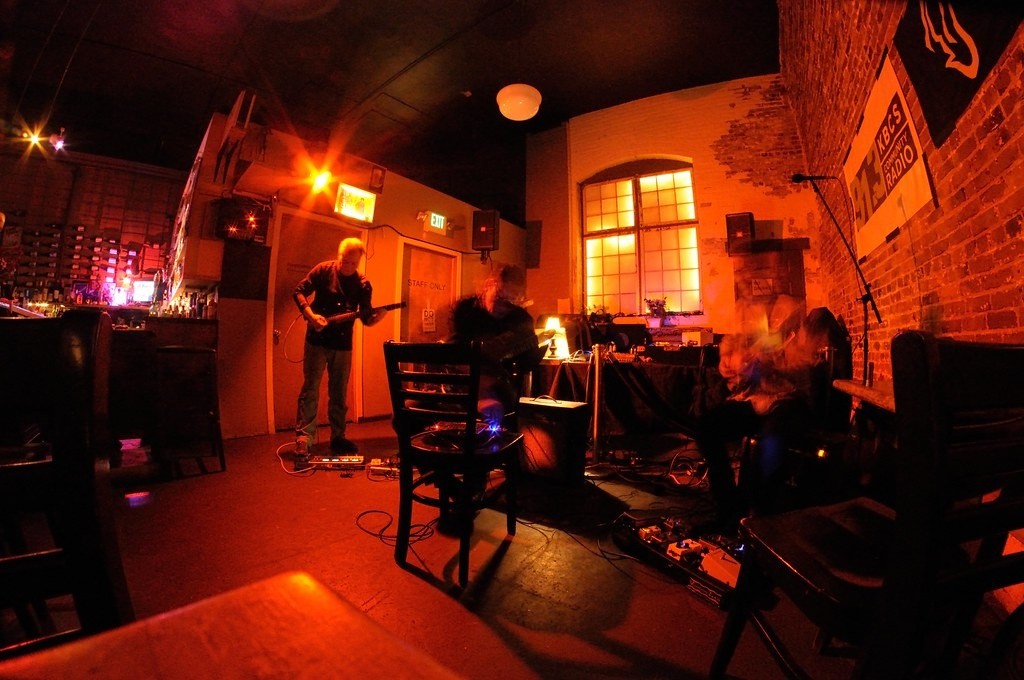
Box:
[331,437,357,453]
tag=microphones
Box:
[792,173,837,184]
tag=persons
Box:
[696,241,819,520]
[292,238,389,451]
[392,263,547,514]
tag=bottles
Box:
[42,284,49,301]
[51,284,60,302]
[149,298,217,319]
[76,292,83,304]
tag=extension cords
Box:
[370,466,399,476]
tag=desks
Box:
[831,377,895,414]
[112,327,156,451]
[0,572,465,680]
[531,358,707,460]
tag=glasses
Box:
[492,288,515,304]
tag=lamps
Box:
[544,317,565,365]
[417,209,455,235]
[496,83,543,121]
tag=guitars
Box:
[498,330,555,362]
[310,297,408,341]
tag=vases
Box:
[646,316,663,328]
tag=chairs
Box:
[150,345,226,482]
[709,330,1024,680]
[384,337,524,587]
[0,306,135,659]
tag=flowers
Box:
[645,298,667,317]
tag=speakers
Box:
[518,395,591,478]
[725,212,755,256]
[471,208,499,251]
